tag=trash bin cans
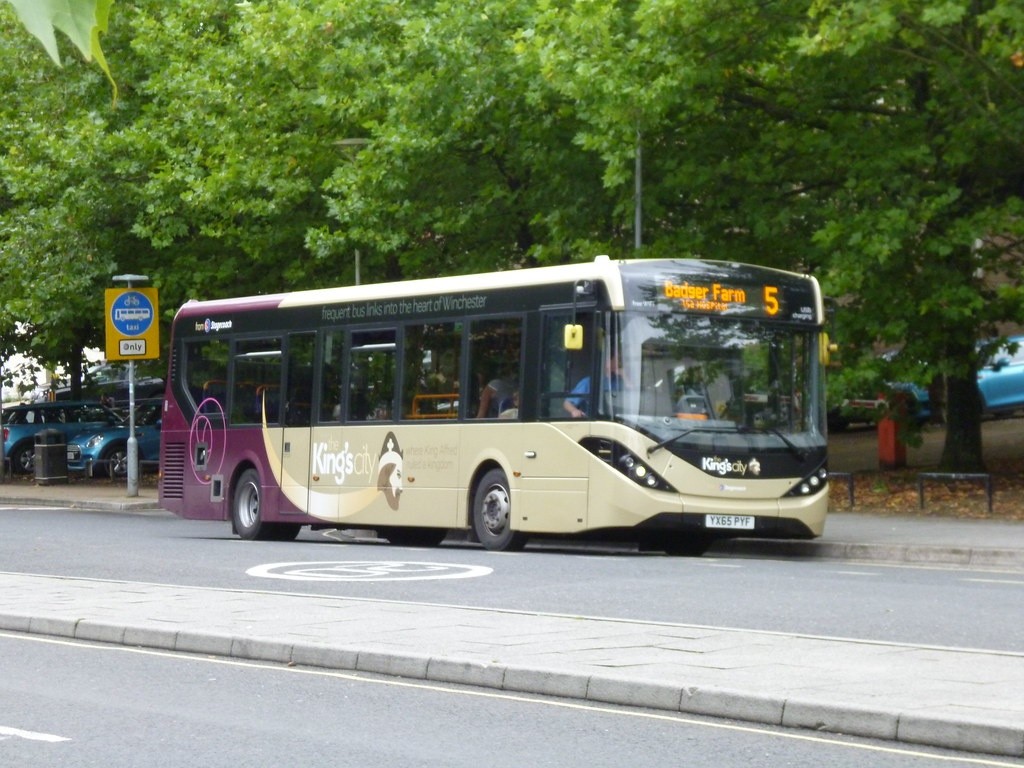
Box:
[33,427,70,485]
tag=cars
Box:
[842,335,1023,427]
[66,398,163,479]
[2,400,124,476]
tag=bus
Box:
[158,254,838,552]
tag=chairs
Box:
[499,396,511,418]
[202,378,408,420]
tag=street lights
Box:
[331,138,373,286]
[114,273,149,498]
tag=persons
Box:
[563,353,624,418]
[101,393,112,408]
[476,378,519,419]
